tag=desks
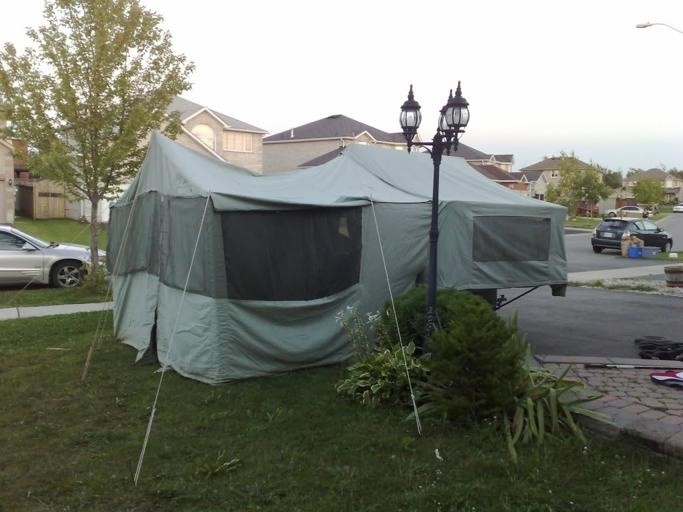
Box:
[396,81,471,352]
[635,20,683,36]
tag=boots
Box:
[573,200,599,217]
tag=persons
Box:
[642,208,649,220]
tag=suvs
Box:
[664,265,683,288]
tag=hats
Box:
[606,206,653,218]
[672,203,682,213]
[589,216,673,255]
[0,221,107,292]
